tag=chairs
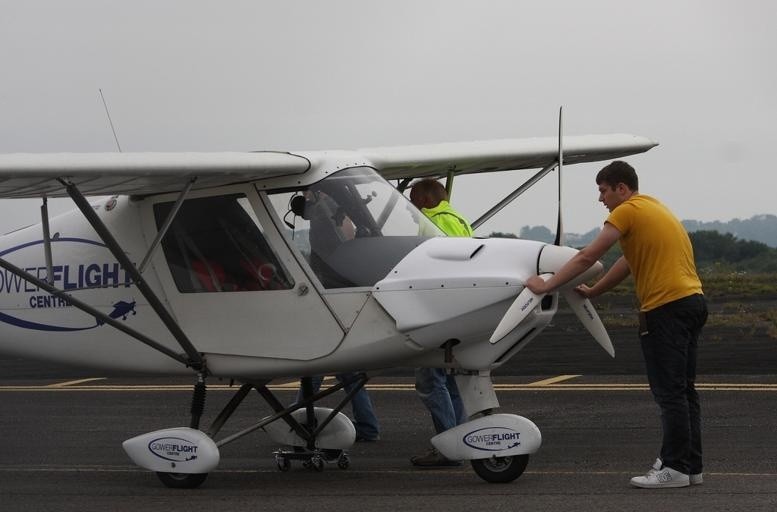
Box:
[177,213,292,291]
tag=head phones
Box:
[291,196,313,220]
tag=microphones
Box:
[283,209,295,229]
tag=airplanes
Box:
[0,105,658,490]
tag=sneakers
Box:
[411,447,465,466]
[630,458,704,488]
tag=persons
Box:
[406,179,474,466]
[193,215,286,292]
[519,160,710,490]
[293,191,381,443]
